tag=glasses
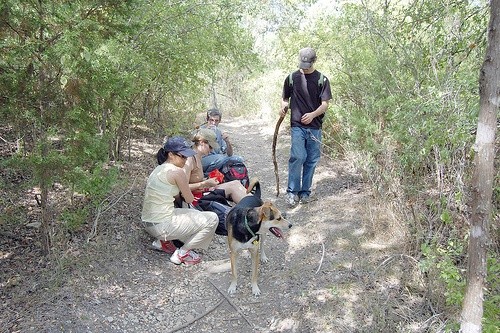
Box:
[175,152,186,159]
[209,144,212,149]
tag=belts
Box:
[142,222,158,227]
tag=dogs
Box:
[223,176,293,299]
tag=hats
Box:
[197,128,220,150]
[299,48,316,69]
[163,136,196,158]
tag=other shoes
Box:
[285,193,298,207]
[299,193,310,203]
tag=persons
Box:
[201,108,242,175]
[182,129,253,204]
[279,48,332,204]
[142,138,219,265]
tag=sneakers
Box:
[170,248,202,266]
[153,239,177,254]
